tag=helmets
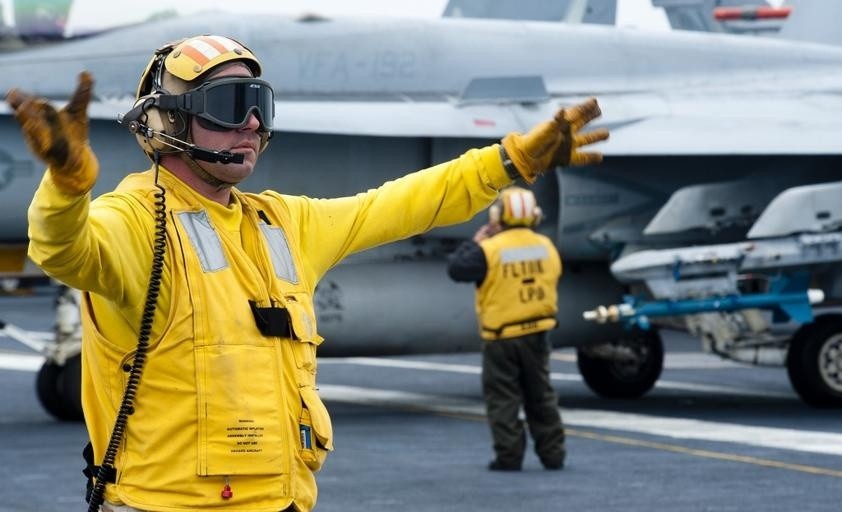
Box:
[496,187,537,227]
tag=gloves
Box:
[500,98,609,186]
[5,70,98,198]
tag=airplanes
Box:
[0,0,842,425]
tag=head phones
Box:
[488,185,545,231]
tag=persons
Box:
[3,33,612,512]
[446,186,567,472]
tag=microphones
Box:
[122,119,248,167]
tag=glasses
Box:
[176,78,276,133]
